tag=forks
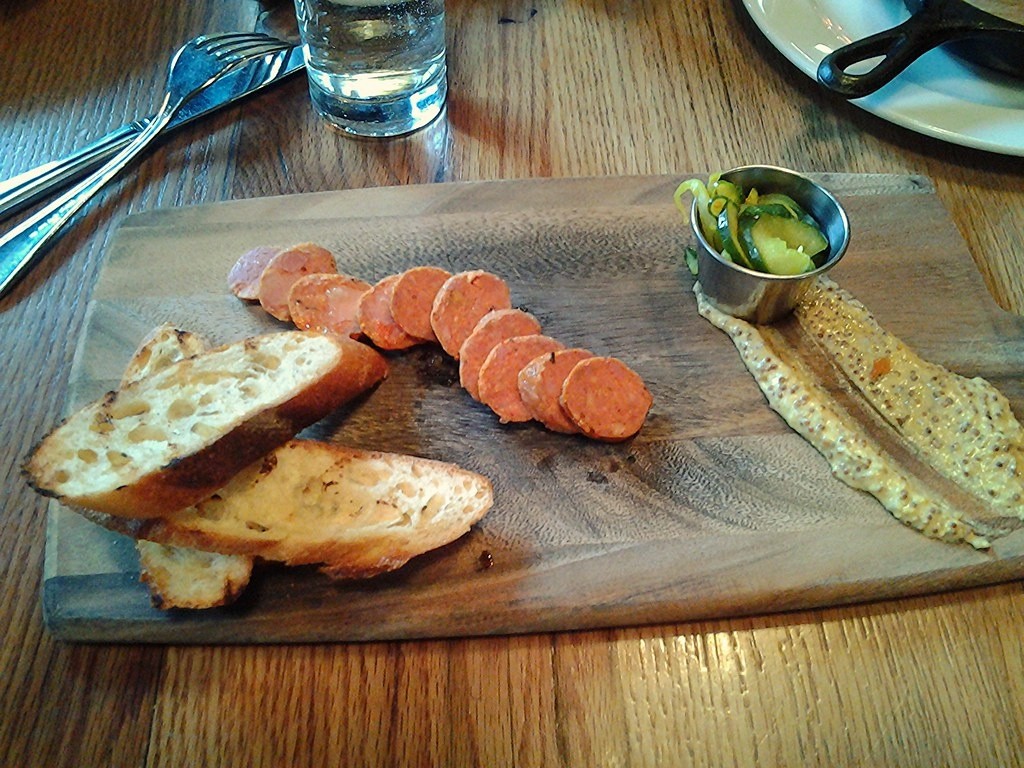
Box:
[0,32,297,299]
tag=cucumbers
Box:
[710,192,829,275]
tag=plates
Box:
[741,0,1024,157]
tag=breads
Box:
[117,322,254,609]
[62,435,495,581]
[18,330,388,520]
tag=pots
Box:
[804,0,1024,100]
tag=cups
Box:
[689,164,851,327]
[292,0,449,140]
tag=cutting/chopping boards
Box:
[39,170,1024,645]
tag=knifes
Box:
[0,45,310,225]
